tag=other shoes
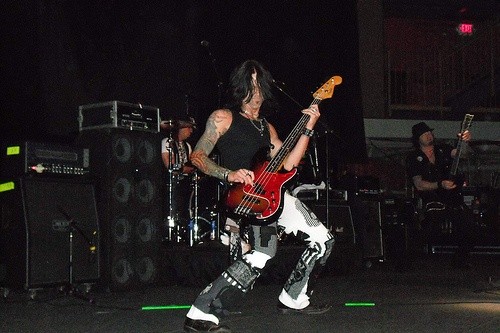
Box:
[184,316,230,333]
[277,299,331,314]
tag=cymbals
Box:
[161,120,197,129]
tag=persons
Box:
[182,59,335,333]
[403,120,475,191]
[161,114,196,207]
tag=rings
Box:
[245,174,248,177]
[312,109,317,112]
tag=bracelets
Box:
[302,128,314,137]
[436,179,444,190]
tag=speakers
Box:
[0,174,101,288]
[78,126,170,295]
[306,204,355,247]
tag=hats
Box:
[412,122,434,139]
[173,117,196,131]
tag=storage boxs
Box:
[77,101,162,133]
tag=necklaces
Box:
[244,111,264,137]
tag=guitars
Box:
[422,114,475,219]
[216,75,342,226]
